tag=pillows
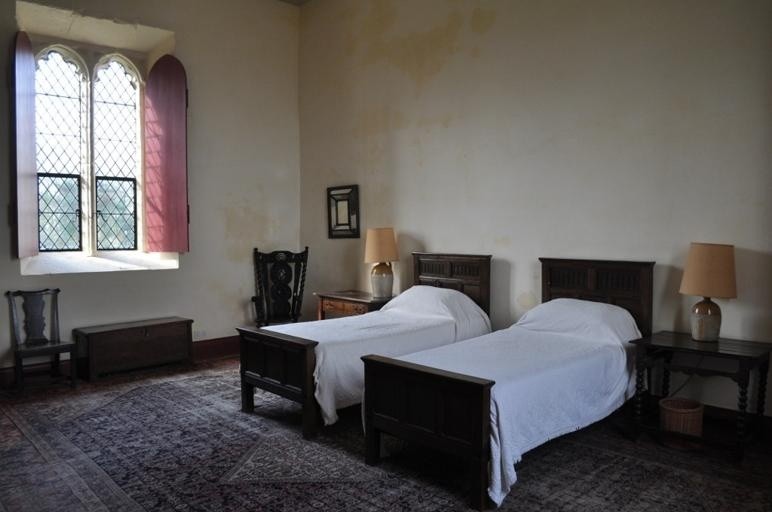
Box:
[380,284,475,320]
[520,297,633,346]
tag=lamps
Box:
[364,227,399,298]
[678,242,738,343]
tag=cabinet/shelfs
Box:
[71,314,194,384]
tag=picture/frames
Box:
[326,184,360,238]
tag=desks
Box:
[628,329,772,468]
[311,289,396,320]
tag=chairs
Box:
[4,287,77,398]
[250,246,308,328]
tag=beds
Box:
[235,251,493,440]
[362,256,656,512]
